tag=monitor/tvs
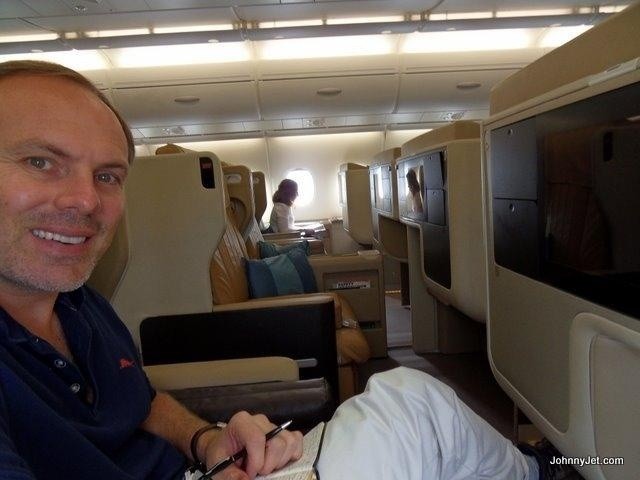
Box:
[534,81,640,324]
[373,173,384,210]
[340,173,346,203]
[404,157,425,220]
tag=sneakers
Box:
[517,437,575,480]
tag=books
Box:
[190,419,326,480]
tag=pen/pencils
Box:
[197,420,292,480]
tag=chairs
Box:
[152,146,372,402]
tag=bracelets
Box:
[190,419,227,472]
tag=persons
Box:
[0,58,576,480]
[404,168,423,214]
[269,178,315,236]
